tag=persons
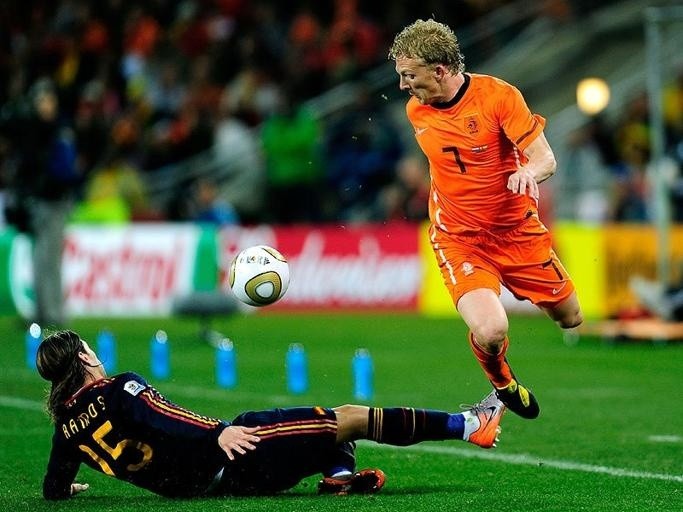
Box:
[35,331,507,498]
[16,77,76,327]
[392,17,580,422]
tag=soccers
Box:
[228,245,289,305]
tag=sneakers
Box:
[489,357,539,419]
[460,389,506,448]
[318,469,385,495]
